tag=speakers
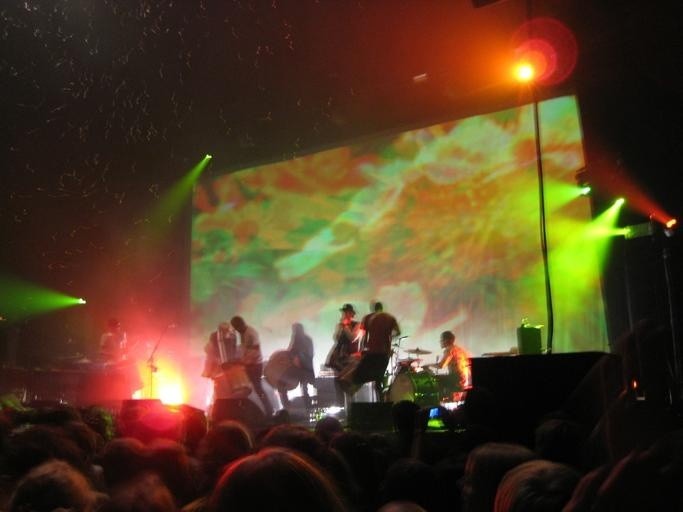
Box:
[212,398,267,428]
[350,402,394,433]
[31,399,62,409]
[121,399,170,421]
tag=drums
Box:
[386,371,443,409]
[382,371,395,393]
[106,359,143,393]
[397,358,423,368]
[264,350,300,390]
[215,359,252,397]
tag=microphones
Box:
[167,324,176,329]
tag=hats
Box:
[339,303,356,316]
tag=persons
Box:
[98,317,128,361]
[0,397,682,511]
[203,298,473,415]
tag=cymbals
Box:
[403,349,431,354]
[390,343,405,350]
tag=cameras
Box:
[428,406,440,419]
